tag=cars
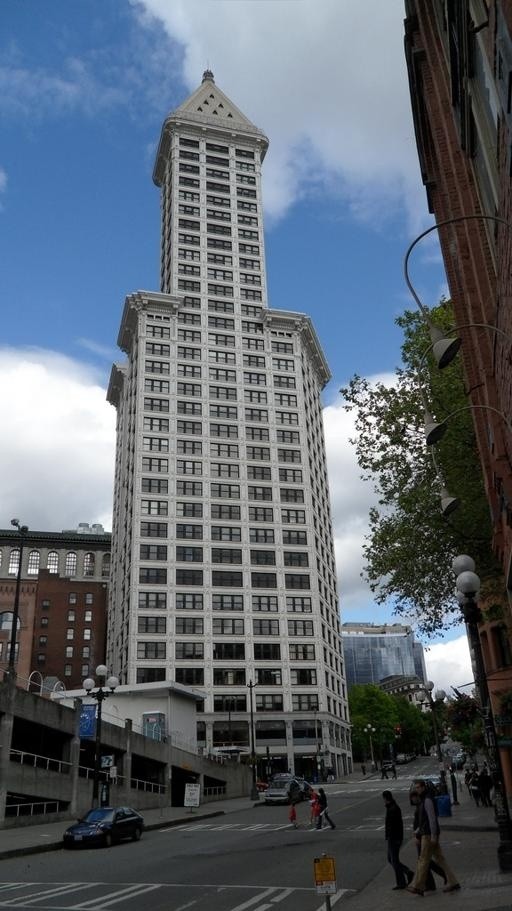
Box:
[382,760,395,771]
[409,775,446,805]
[256,773,314,804]
[451,748,466,769]
[63,806,144,848]
[395,752,416,764]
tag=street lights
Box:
[417,681,447,795]
[4,518,28,680]
[364,723,376,772]
[452,554,512,873]
[83,664,118,810]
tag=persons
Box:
[289,802,296,823]
[307,788,319,825]
[380,766,389,779]
[316,788,336,830]
[405,756,490,895]
[391,762,397,780]
[360,764,366,775]
[382,790,414,890]
[312,800,319,825]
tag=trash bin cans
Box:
[437,794,451,817]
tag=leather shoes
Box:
[392,871,436,896]
[443,880,461,892]
